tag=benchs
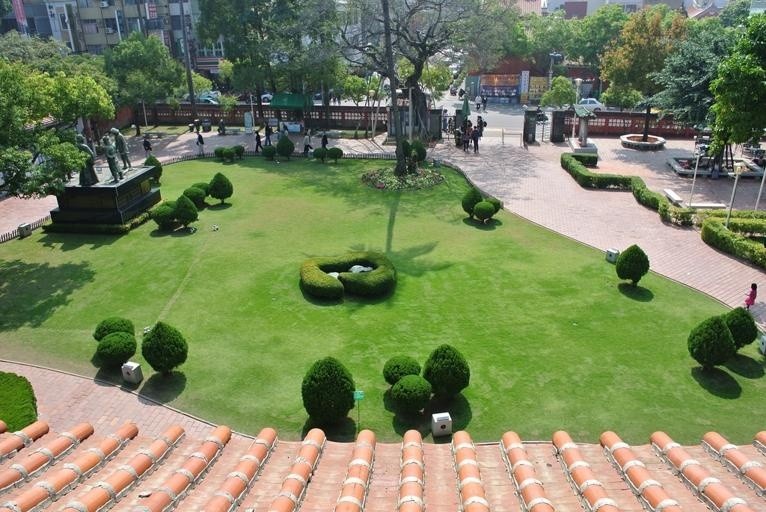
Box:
[664,189,726,210]
[143,131,167,138]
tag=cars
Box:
[440,48,469,79]
[182,87,292,105]
[556,98,606,113]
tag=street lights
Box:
[725,161,748,228]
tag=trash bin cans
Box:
[19,224,31,237]
[432,411,452,437]
[122,361,143,384]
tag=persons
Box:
[195,131,204,157]
[442,83,490,153]
[142,137,153,158]
[744,283,757,311]
[254,122,329,153]
[218,117,226,135]
[102,136,124,183]
[110,127,133,171]
[75,133,99,186]
